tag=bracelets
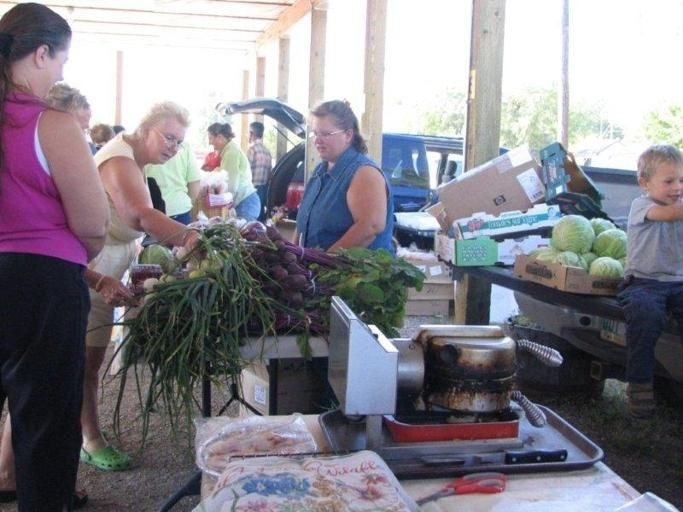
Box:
[95,273,107,291]
[178,228,192,247]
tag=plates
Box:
[194,428,317,478]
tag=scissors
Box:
[418,472,506,506]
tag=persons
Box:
[138,143,201,225]
[2,83,90,502]
[245,122,272,221]
[77,101,190,472]
[88,122,114,155]
[616,144,683,418]
[208,122,261,222]
[1,2,109,508]
[290,101,397,259]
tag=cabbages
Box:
[594,229,629,258]
[620,256,626,265]
[582,251,598,263]
[140,243,176,275]
[537,248,561,266]
[590,257,624,278]
[553,251,587,274]
[551,213,595,253]
[591,217,616,233]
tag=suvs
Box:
[214,98,516,250]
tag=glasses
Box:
[152,126,186,153]
[311,128,346,138]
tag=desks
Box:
[120,271,330,512]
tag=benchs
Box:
[442,256,683,340]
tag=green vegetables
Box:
[104,278,261,429]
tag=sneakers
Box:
[625,378,661,416]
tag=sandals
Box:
[81,441,135,473]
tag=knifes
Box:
[418,450,569,464]
